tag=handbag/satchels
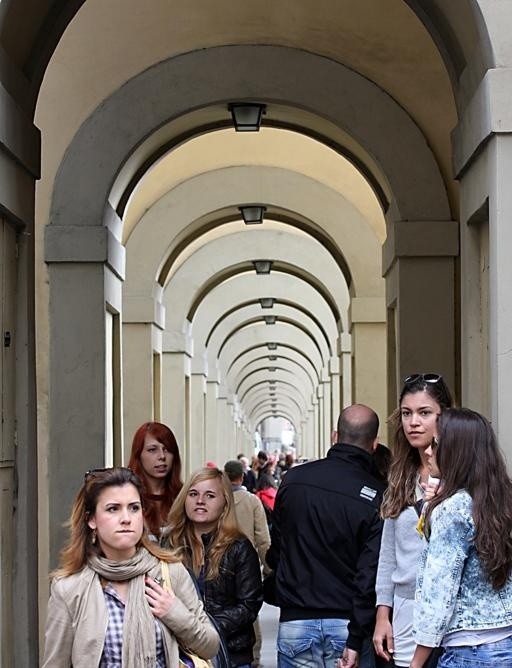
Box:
[179,647,214,668]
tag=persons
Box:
[160,468,264,667]
[273,404,389,667]
[207,449,304,607]
[373,373,455,667]
[410,408,512,668]
[128,422,185,546]
[224,461,272,667]
[42,468,220,668]
[372,444,397,666]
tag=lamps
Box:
[226,101,269,131]
[263,316,277,324]
[252,261,273,275]
[237,205,266,224]
[258,298,275,308]
[267,343,279,418]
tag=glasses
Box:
[85,467,132,477]
[431,437,440,450]
[404,374,442,383]
